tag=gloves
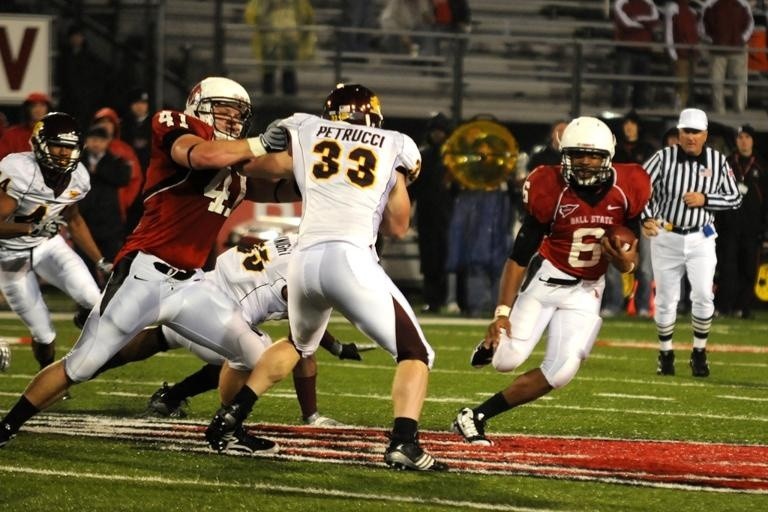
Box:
[304,412,346,426]
[262,118,290,154]
[335,340,377,361]
[30,215,68,239]
[96,257,116,290]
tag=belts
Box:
[152,262,196,281]
[660,223,700,235]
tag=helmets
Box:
[31,110,84,174]
[559,114,617,194]
[184,76,254,140]
[320,83,383,128]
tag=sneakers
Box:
[689,346,710,377]
[383,437,449,471]
[206,406,280,455]
[470,337,493,368]
[147,382,186,418]
[450,406,494,447]
[0,421,17,446]
[656,350,675,376]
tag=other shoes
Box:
[447,303,460,316]
[638,309,649,319]
[602,310,616,318]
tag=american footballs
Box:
[608,223,635,250]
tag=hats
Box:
[677,108,708,131]
[734,123,758,142]
[86,126,110,138]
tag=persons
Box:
[638,108,744,377]
[246,1,316,94]
[442,114,529,317]
[610,0,659,110]
[0,75,281,459]
[0,114,114,400]
[698,0,755,114]
[0,13,155,290]
[454,115,651,449]
[665,0,701,109]
[525,109,767,320]
[403,112,454,313]
[219,80,452,476]
[54,228,385,432]
[343,1,470,96]
